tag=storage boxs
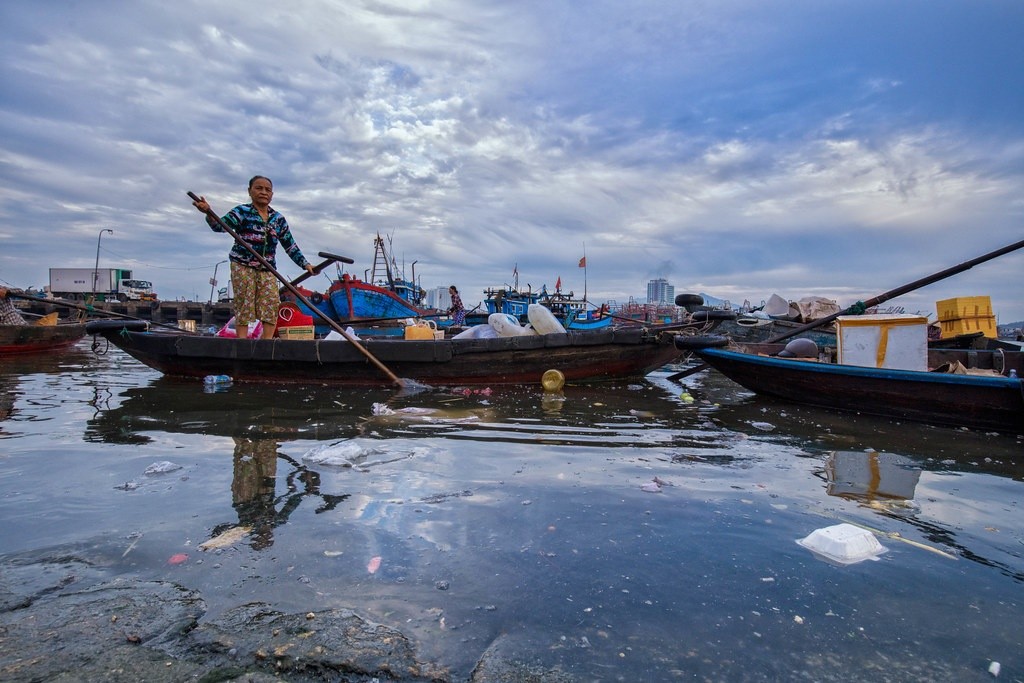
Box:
[936,296,994,322]
[277,326,316,340]
[940,316,997,340]
[835,314,928,372]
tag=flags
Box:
[579,257,585,267]
[556,277,561,288]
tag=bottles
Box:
[205,375,233,385]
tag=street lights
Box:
[210,260,228,302]
[91,228,114,305]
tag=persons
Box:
[0,284,29,326]
[447,285,465,326]
[193,176,321,338]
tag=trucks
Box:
[48,267,154,302]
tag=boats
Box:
[0,307,87,350]
[82,310,698,384]
[273,228,618,331]
[691,330,1024,436]
